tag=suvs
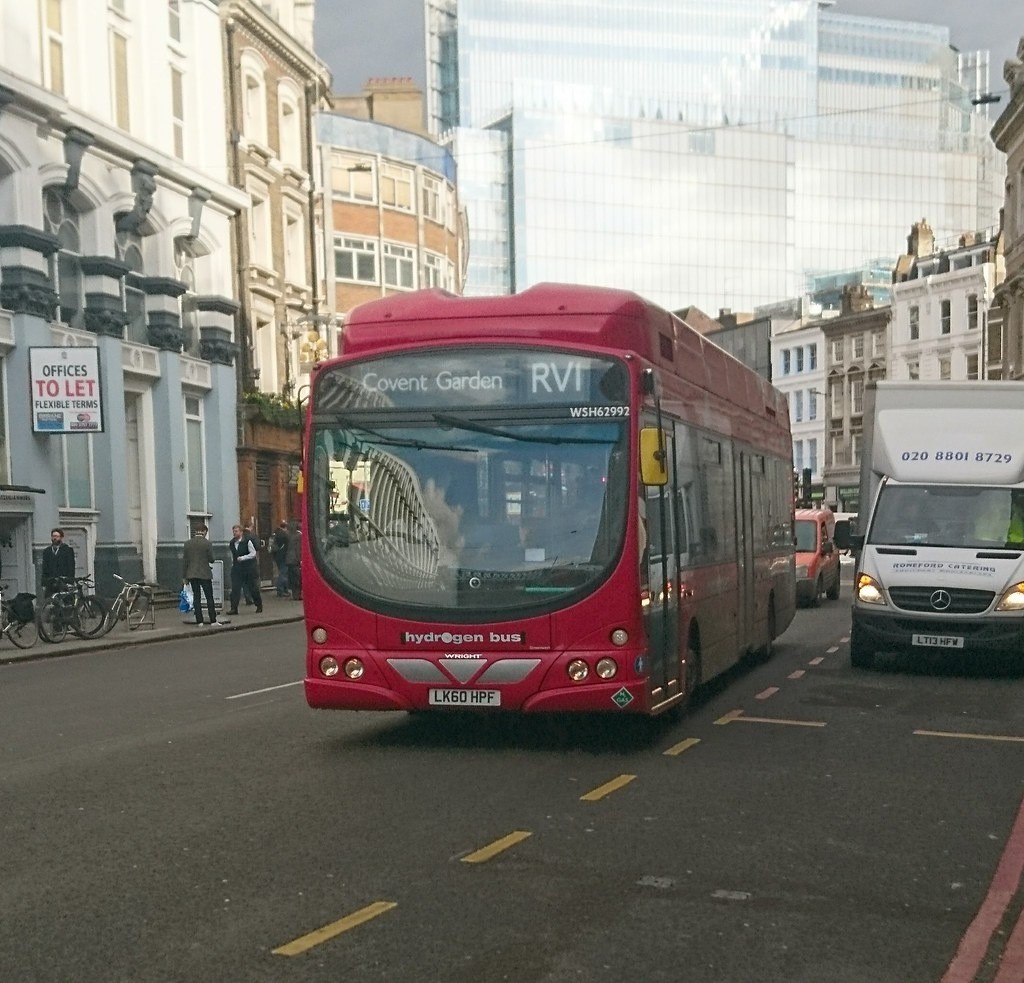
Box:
[794,508,841,607]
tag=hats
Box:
[196,522,208,531]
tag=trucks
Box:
[849,378,1024,670]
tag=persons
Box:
[41,528,74,632]
[226,522,302,614]
[181,525,223,627]
[895,502,942,546]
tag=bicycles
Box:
[102,574,152,634]
[0,583,38,650]
[34,573,116,644]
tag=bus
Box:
[297,281,798,726]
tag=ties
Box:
[52,546,58,552]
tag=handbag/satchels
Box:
[269,544,280,553]
[178,582,195,614]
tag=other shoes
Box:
[255,605,263,613]
[197,623,204,627]
[210,621,222,628]
[225,607,239,615]
[276,591,291,597]
